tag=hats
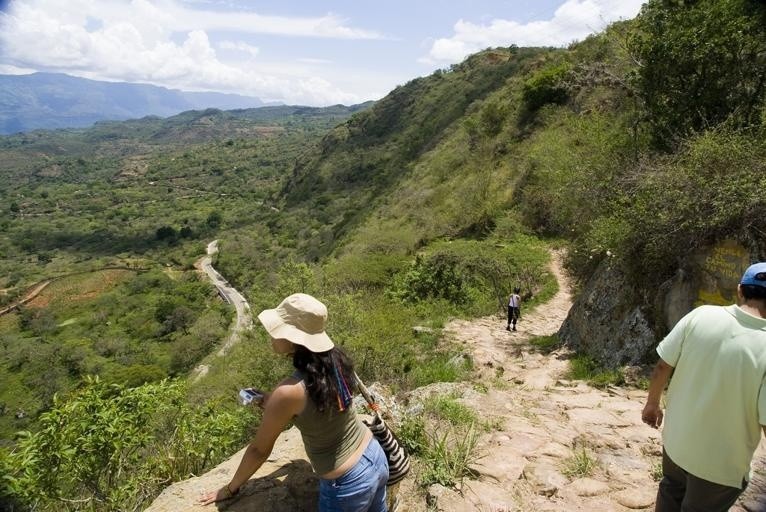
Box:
[740,263,766,287]
[257,294,334,353]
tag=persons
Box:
[199,293,389,512]
[642,262,766,512]
[506,287,522,330]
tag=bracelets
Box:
[224,483,239,498]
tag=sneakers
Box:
[507,326,516,331]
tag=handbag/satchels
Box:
[363,412,410,485]
[513,309,520,316]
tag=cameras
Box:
[238,387,264,407]
